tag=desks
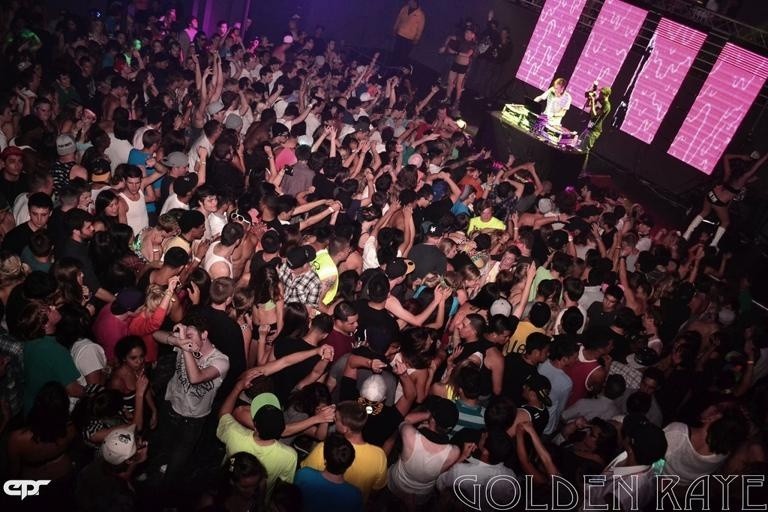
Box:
[483,110,589,195]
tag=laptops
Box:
[523,95,544,115]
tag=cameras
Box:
[585,79,599,98]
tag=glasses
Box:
[187,343,202,359]
[135,440,149,451]
[230,212,251,226]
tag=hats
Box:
[360,91,375,102]
[89,158,111,182]
[172,171,198,196]
[205,101,230,116]
[578,204,604,218]
[54,132,77,156]
[460,183,476,201]
[283,34,294,42]
[271,121,289,137]
[620,411,668,467]
[249,392,286,441]
[160,151,189,168]
[358,373,387,402]
[224,113,243,131]
[426,222,441,237]
[489,295,512,318]
[430,181,451,202]
[537,197,553,213]
[384,257,416,280]
[406,153,428,169]
[284,244,316,270]
[422,394,459,431]
[523,373,552,408]
[99,422,138,466]
[2,145,23,157]
